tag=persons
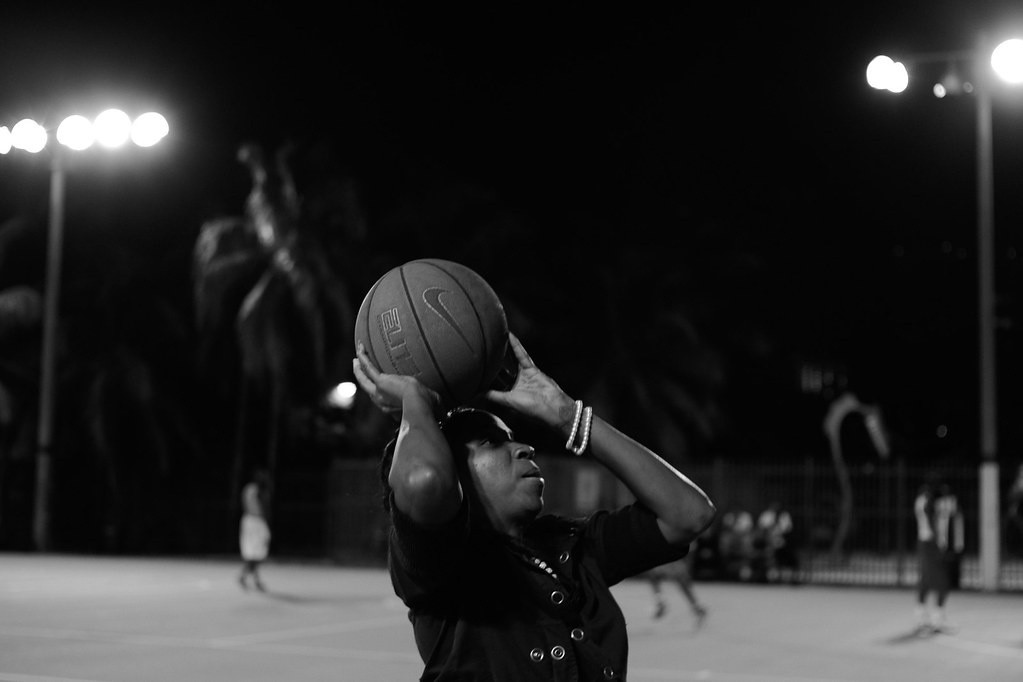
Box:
[756,501,801,581]
[354,330,719,682]
[646,554,709,630]
[722,503,753,581]
[235,469,271,591]
[916,467,966,636]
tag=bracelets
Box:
[565,399,582,448]
[574,406,591,455]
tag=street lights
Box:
[0,108,171,551]
[865,34,1023,597]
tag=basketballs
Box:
[353,259,509,412]
